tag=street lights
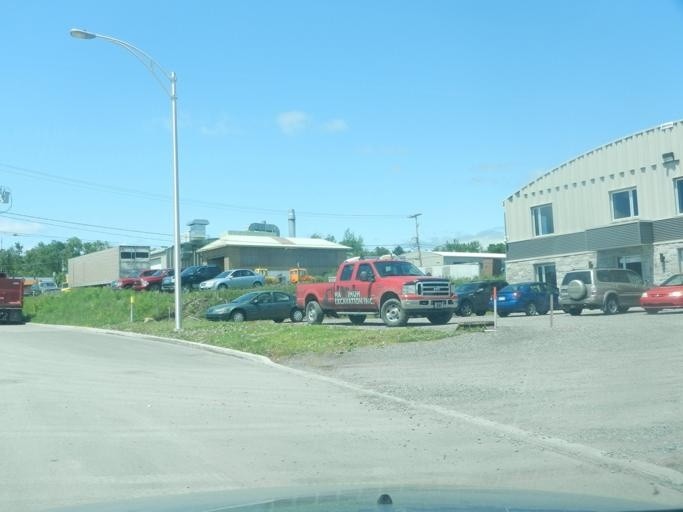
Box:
[70,29,182,331]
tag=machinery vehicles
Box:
[255,267,281,285]
[287,262,314,284]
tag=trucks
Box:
[0,272,25,323]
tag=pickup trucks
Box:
[31,281,59,296]
[294,257,462,327]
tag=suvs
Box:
[557,268,660,316]
[454,279,507,318]
[110,264,222,292]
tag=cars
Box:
[58,283,71,293]
[639,273,682,316]
[206,290,305,323]
[488,280,567,318]
[198,269,264,292]
